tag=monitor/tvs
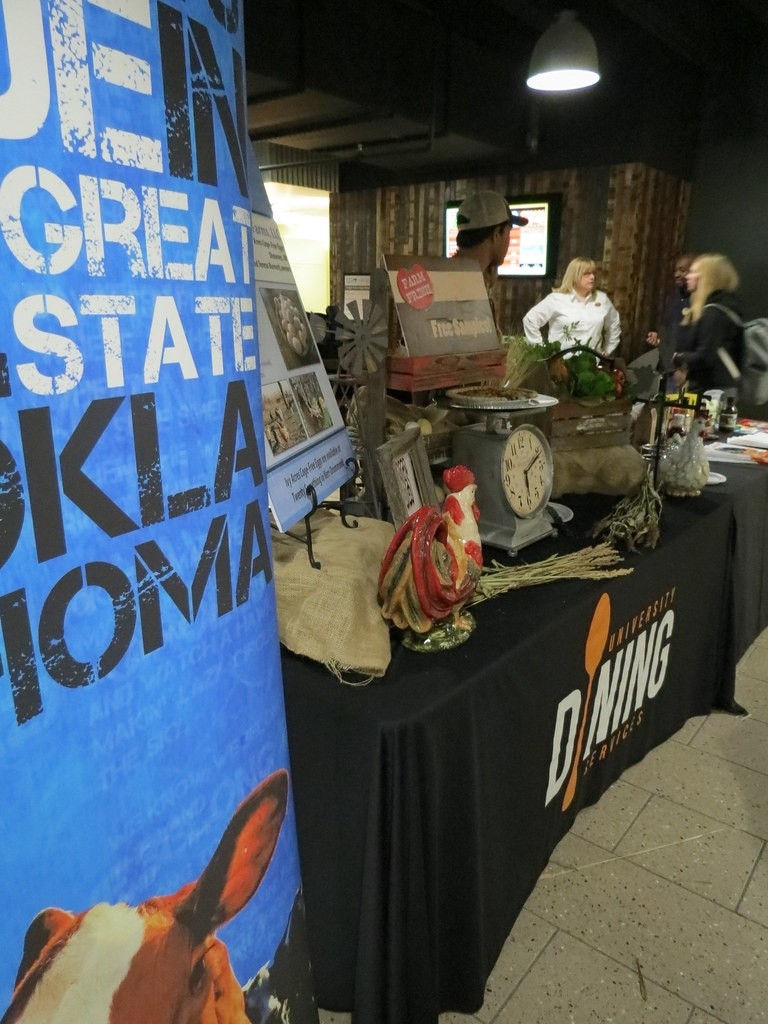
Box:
[445,192,559,280]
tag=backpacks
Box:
[705,302,768,417]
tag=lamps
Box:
[501,422,555,520]
[526,10,600,91]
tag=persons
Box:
[672,253,742,411]
[452,191,528,342]
[736,318,768,421]
[394,458,414,508]
[646,253,699,394]
[522,256,622,360]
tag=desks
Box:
[278,404,768,1024]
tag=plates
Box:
[705,472,726,485]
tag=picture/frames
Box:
[375,427,441,532]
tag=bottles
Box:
[719,397,738,432]
[685,413,691,433]
[668,413,686,439]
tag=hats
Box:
[456,189,529,232]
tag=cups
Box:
[642,454,663,472]
[641,444,657,454]
